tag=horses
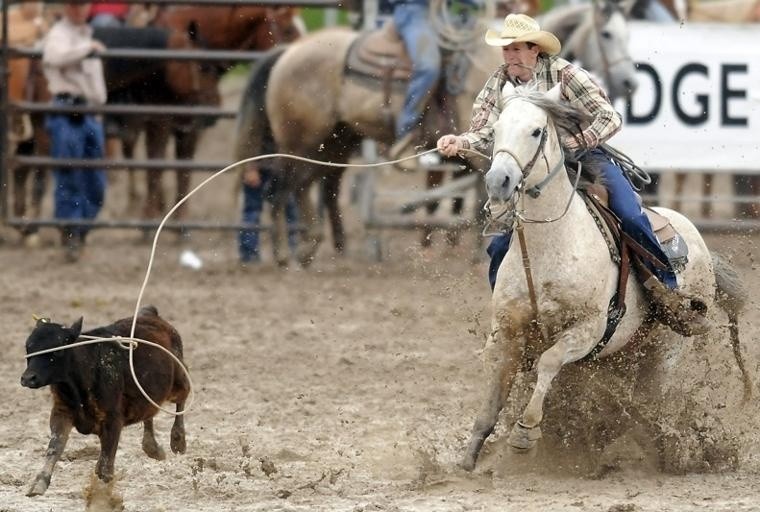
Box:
[231,0,641,275]
[0,1,309,246]
[454,80,751,476]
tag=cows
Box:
[21,304,192,497]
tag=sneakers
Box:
[396,145,420,173]
[656,306,711,336]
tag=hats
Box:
[484,13,561,57]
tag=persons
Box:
[233,161,319,269]
[42,1,111,262]
[628,165,759,227]
[432,7,707,334]
[5,1,62,152]
[384,0,491,169]
